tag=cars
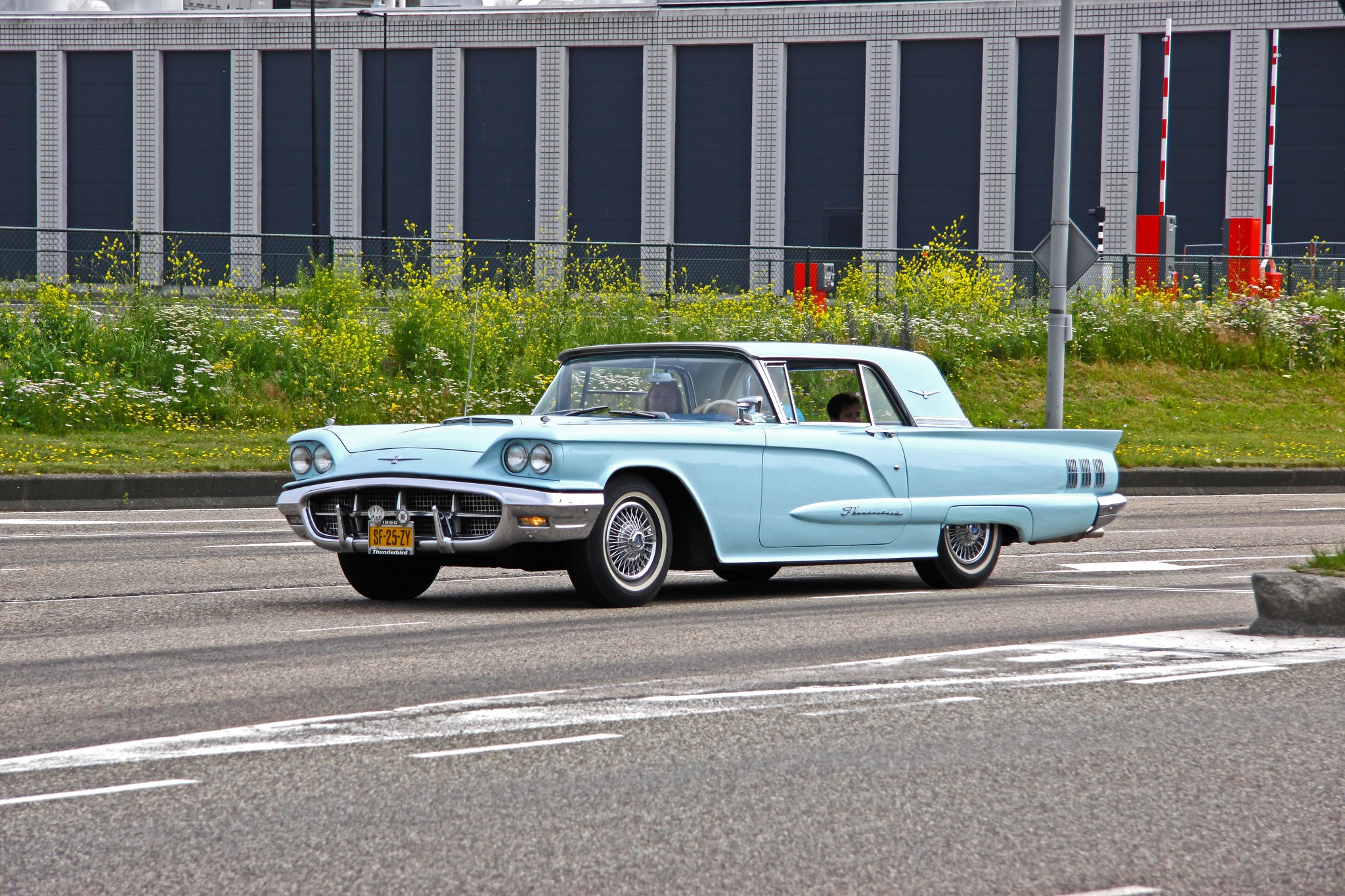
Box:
[273,343,1128,589]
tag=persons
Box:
[825,393,862,422]
[644,380,683,414]
[692,368,805,422]
